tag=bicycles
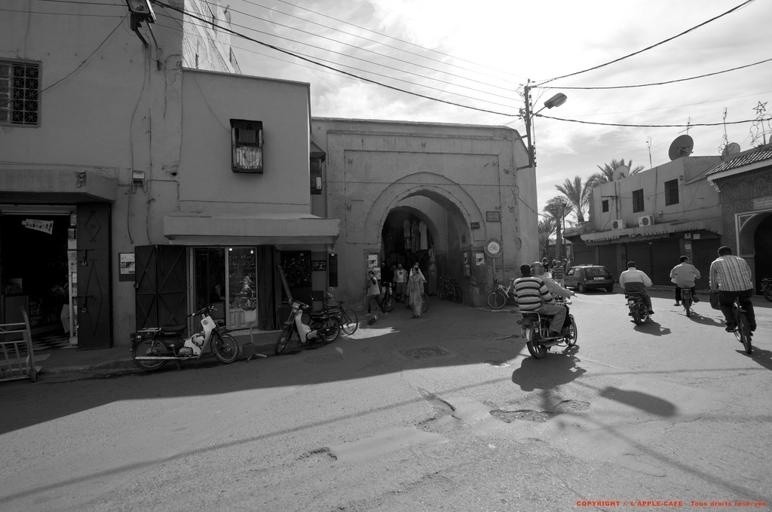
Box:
[310,295,358,338]
[716,286,754,355]
[379,273,462,314]
[484,276,521,309]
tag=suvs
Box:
[559,265,617,293]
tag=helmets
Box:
[530,261,544,275]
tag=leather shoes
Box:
[649,311,654,314]
[726,322,736,331]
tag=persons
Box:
[670,256,701,306]
[619,261,654,315]
[709,246,756,332]
[513,257,571,338]
[214,284,225,302]
[366,262,426,319]
[51,274,69,337]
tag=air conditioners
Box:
[637,214,655,228]
[610,219,626,230]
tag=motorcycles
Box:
[128,301,240,372]
[679,289,696,316]
[760,276,772,303]
[623,284,652,325]
[264,291,340,359]
[507,287,580,359]
